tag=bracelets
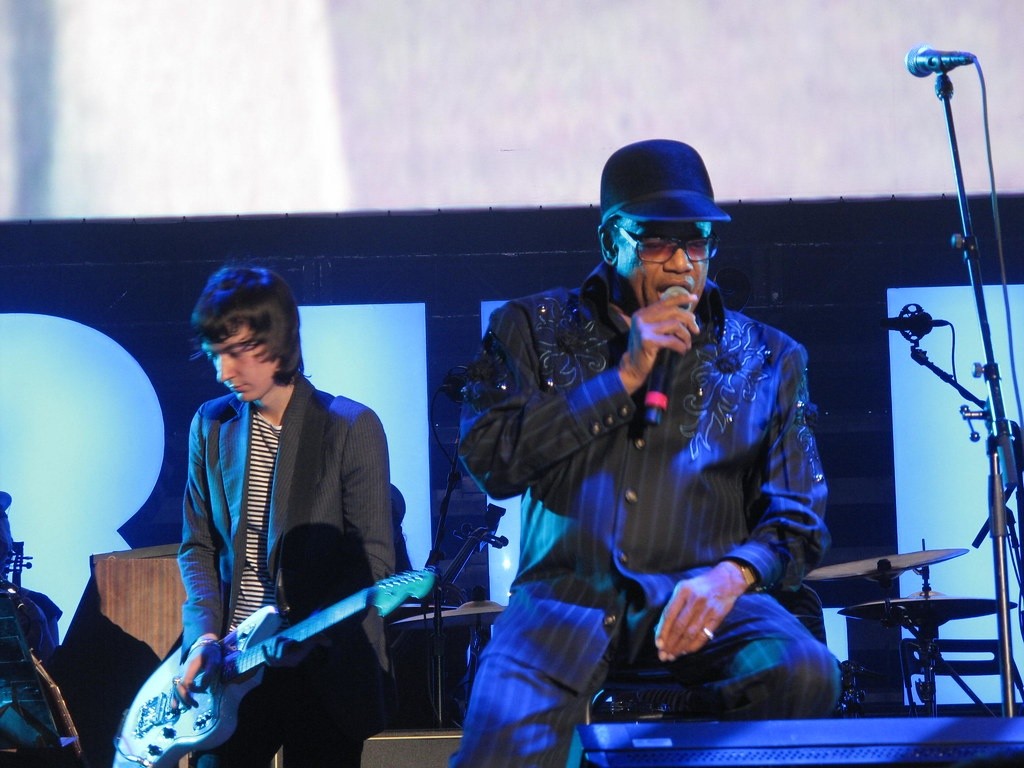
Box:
[740,563,758,588]
[189,638,222,651]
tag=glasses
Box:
[613,220,720,263]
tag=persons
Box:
[446,138,840,768]
[170,264,394,768]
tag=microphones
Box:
[880,303,950,343]
[904,44,975,78]
[643,285,695,424]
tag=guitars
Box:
[111,565,436,768]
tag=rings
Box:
[703,628,714,641]
[172,677,181,685]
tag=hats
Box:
[600,138,732,231]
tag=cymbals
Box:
[837,589,1019,627]
[388,599,508,629]
[803,548,971,583]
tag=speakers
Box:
[564,719,1022,768]
